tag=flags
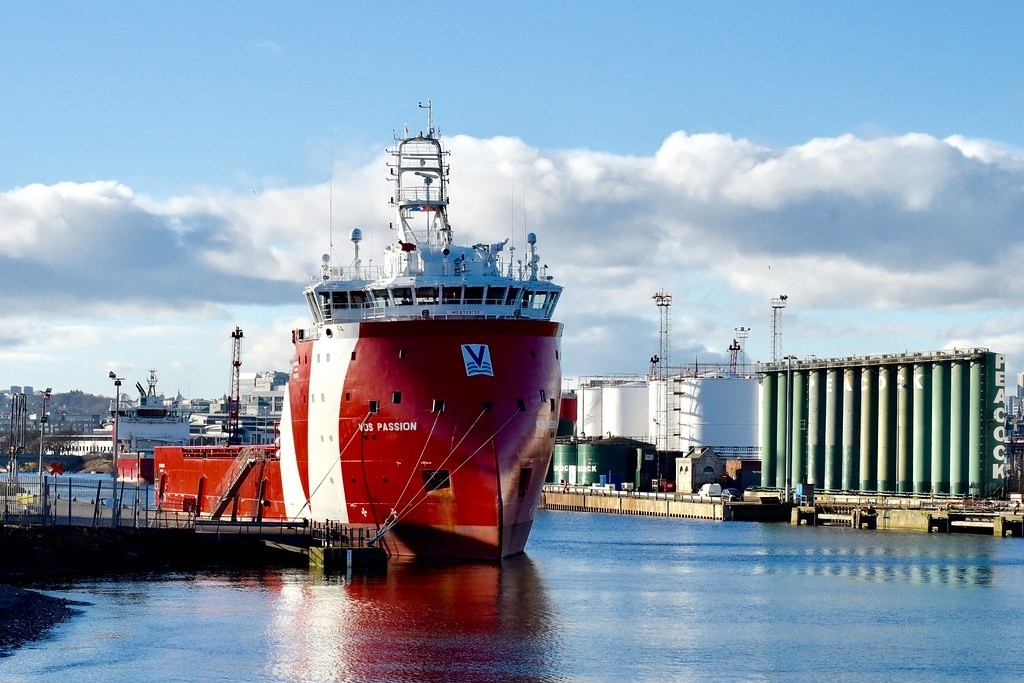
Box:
[421,205,437,211]
[409,205,423,211]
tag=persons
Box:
[1014,500,1021,515]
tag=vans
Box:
[698,483,721,498]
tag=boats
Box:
[153,99,566,562]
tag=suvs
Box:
[721,488,743,502]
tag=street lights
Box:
[14,447,24,493]
[108,371,126,499]
[37,388,52,488]
